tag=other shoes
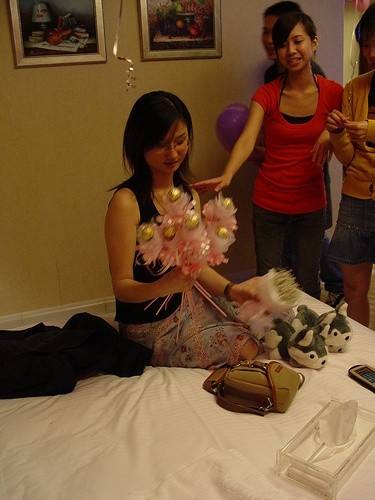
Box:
[326,292,346,311]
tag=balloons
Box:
[217,103,250,152]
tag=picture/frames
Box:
[7,0,109,68]
[138,0,223,61]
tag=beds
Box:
[0,288,375,500]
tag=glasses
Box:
[143,138,190,154]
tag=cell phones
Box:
[348,364,375,393]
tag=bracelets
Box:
[328,126,345,134]
[224,283,236,302]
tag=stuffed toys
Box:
[265,316,330,369]
[291,301,349,353]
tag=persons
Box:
[104,1,375,372]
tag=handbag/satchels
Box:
[203,359,306,417]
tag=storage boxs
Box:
[278,398,375,500]
